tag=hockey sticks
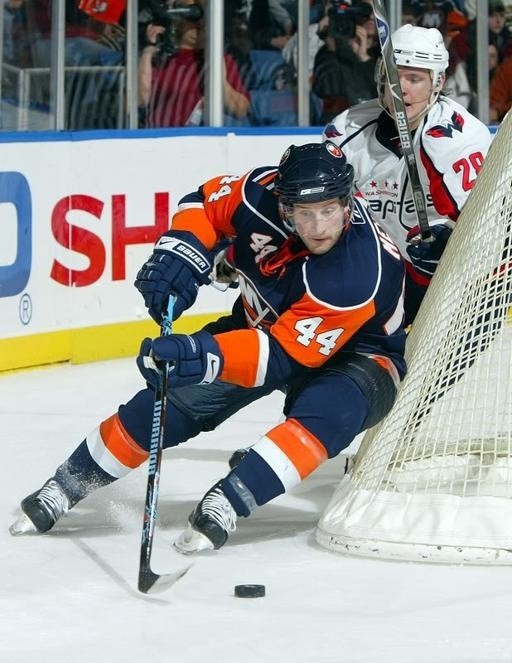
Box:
[138,291,195,594]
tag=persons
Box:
[21,141,409,551]
[0,0,512,129]
[319,24,493,328]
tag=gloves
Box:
[134,231,229,326]
[138,329,223,393]
[406,220,455,281]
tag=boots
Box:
[20,415,151,532]
[189,416,327,548]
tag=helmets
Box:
[275,141,356,234]
[375,26,448,108]
